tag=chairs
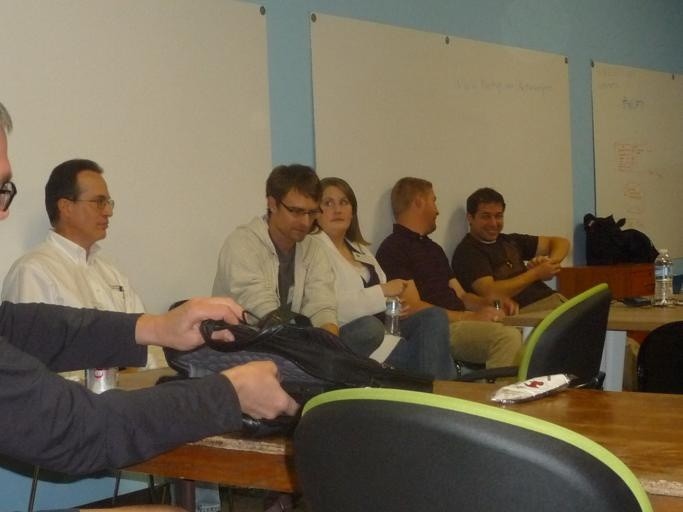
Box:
[292,384,654,512]
[637,320,682,395]
[23,456,174,511]
[452,288,608,388]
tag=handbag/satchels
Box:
[156,300,404,438]
[584,213,621,266]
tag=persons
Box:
[0,104,300,484]
[212,164,339,336]
[4,161,145,379]
[452,188,570,312]
[375,177,522,377]
[310,177,457,380]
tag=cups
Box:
[490,295,503,320]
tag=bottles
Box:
[653,248,674,307]
[385,294,398,333]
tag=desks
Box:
[505,303,682,394]
[117,367,683,512]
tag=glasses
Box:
[272,194,323,219]
[0,181,15,212]
[67,197,115,209]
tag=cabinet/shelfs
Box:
[556,264,655,342]
